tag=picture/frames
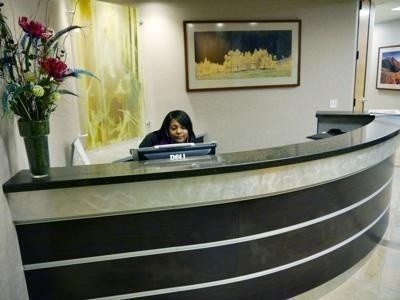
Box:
[182,20,301,92]
[375,45,400,91]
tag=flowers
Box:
[0,1,101,174]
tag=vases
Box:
[17,115,50,180]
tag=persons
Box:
[158,110,197,144]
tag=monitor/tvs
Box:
[130,142,216,162]
